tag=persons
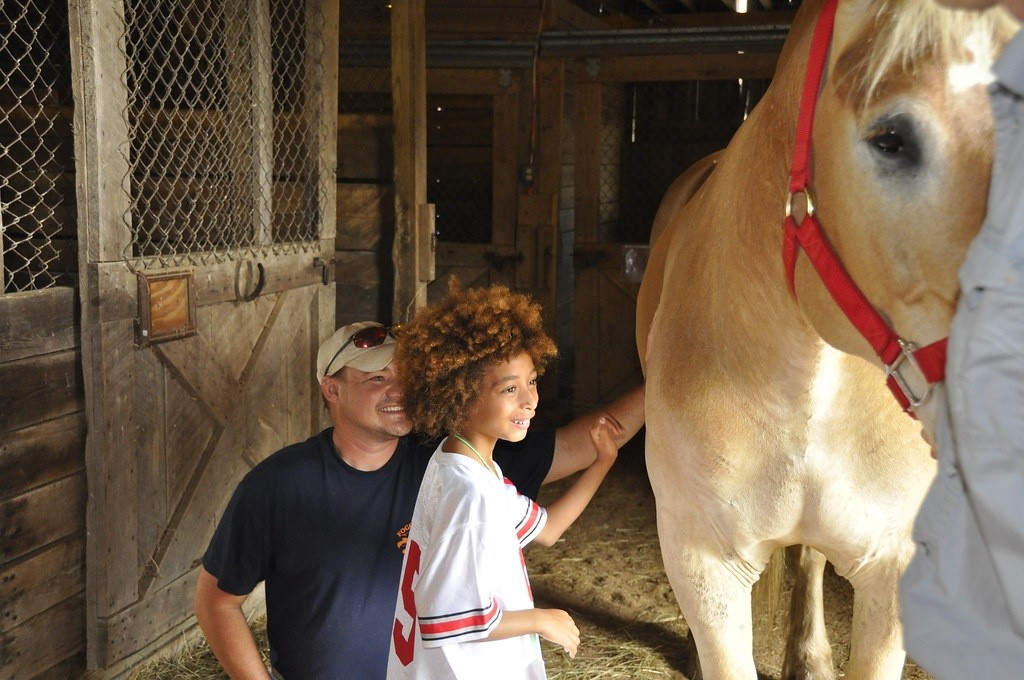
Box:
[193,320,647,680]
[383,273,627,680]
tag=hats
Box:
[316,320,401,385]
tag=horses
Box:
[633,1,1023,680]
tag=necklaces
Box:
[446,430,503,485]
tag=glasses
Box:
[324,325,397,375]
